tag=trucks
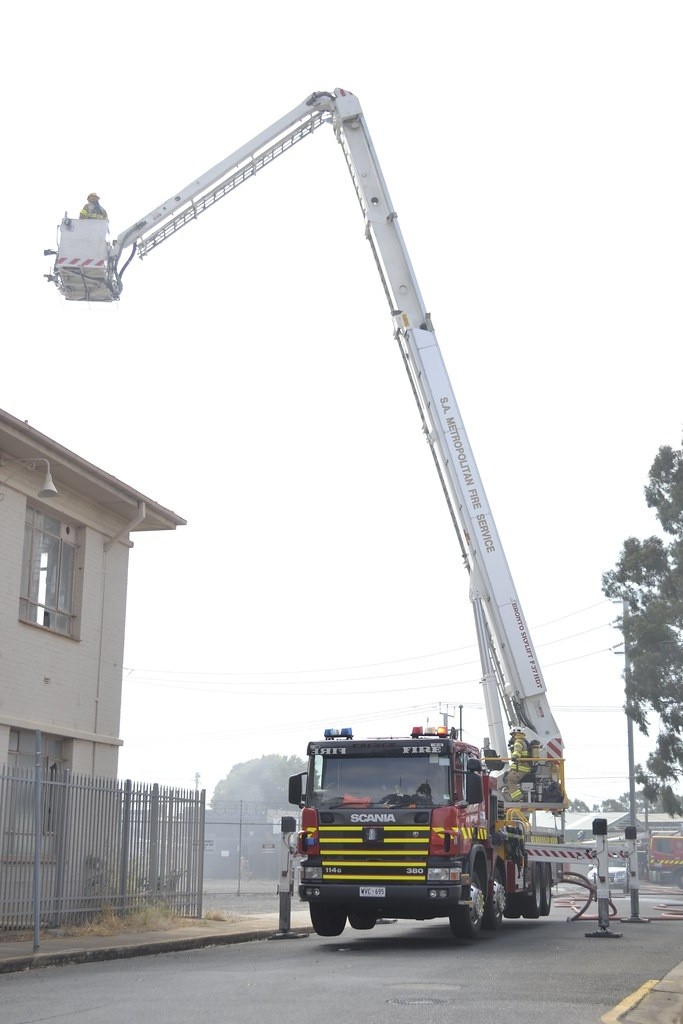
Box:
[647,835,683,890]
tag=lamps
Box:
[0,455,59,501]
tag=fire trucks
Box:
[43,88,651,938]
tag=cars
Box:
[586,854,629,894]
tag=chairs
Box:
[142,866,188,905]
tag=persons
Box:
[80,193,108,221]
[506,732,531,802]
